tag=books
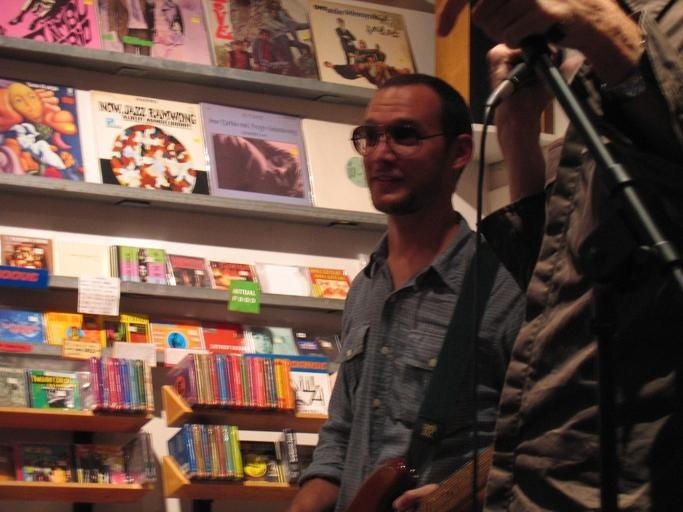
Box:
[1,236,53,274]
[1,304,343,485]
[1,79,387,214]
[1,2,415,89]
[109,246,352,301]
[359,255,370,267]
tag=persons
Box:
[286,70,529,511]
[435,0,681,512]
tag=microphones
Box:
[484,42,564,109]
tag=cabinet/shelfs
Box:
[0,1,562,511]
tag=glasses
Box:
[350,126,462,156]
